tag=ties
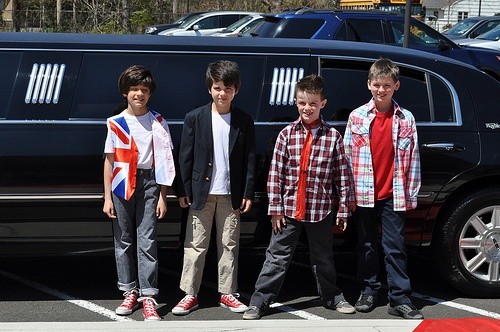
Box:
[294,118,321,221]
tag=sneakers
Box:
[116,289,140,315]
[388,302,424,319]
[137,295,161,321]
[355,290,375,312]
[332,293,355,313]
[218,293,248,313]
[242,305,265,319]
[172,295,198,315]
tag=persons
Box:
[101,63,174,321]
[172,58,256,314]
[343,59,426,322]
[240,71,357,320]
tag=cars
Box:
[144,5,500,82]
[1,31,500,301]
